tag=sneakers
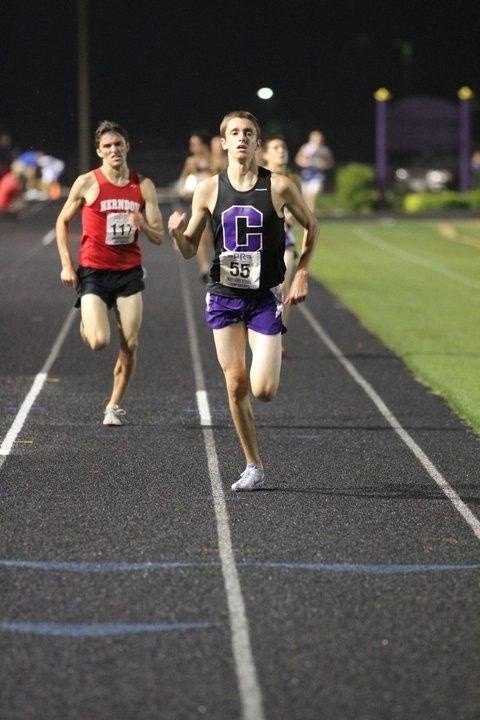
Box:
[103,405,126,426]
[231,465,266,492]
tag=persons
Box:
[55,120,166,426]
[177,130,332,355]
[168,110,321,490]
